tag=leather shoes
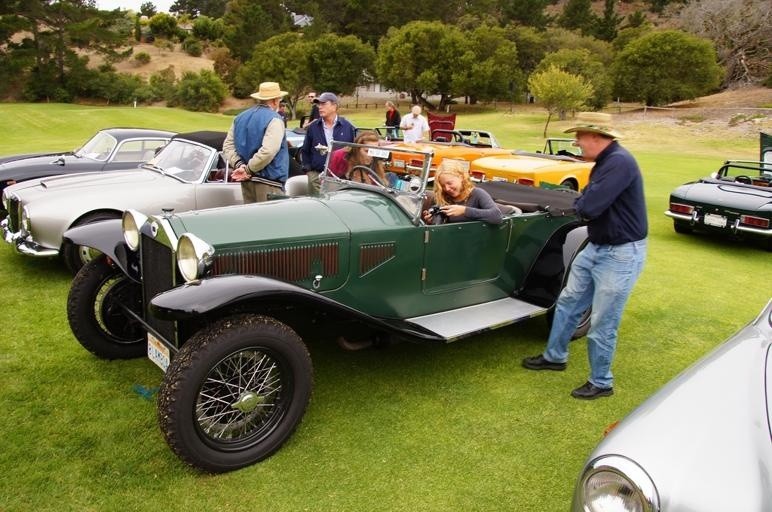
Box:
[523,354,566,370]
[572,381,613,399]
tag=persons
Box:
[422,159,504,225]
[384,102,400,138]
[302,94,356,196]
[278,103,288,127]
[399,106,429,143]
[303,90,319,130]
[326,129,389,185]
[522,112,649,401]
[223,82,289,204]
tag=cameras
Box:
[429,205,445,215]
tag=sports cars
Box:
[668,155,767,253]
[1,132,312,275]
[1,129,182,212]
[376,129,606,197]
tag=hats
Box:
[250,82,288,100]
[314,92,337,103]
[563,111,624,138]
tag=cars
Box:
[570,295,771,507]
[62,178,613,474]
